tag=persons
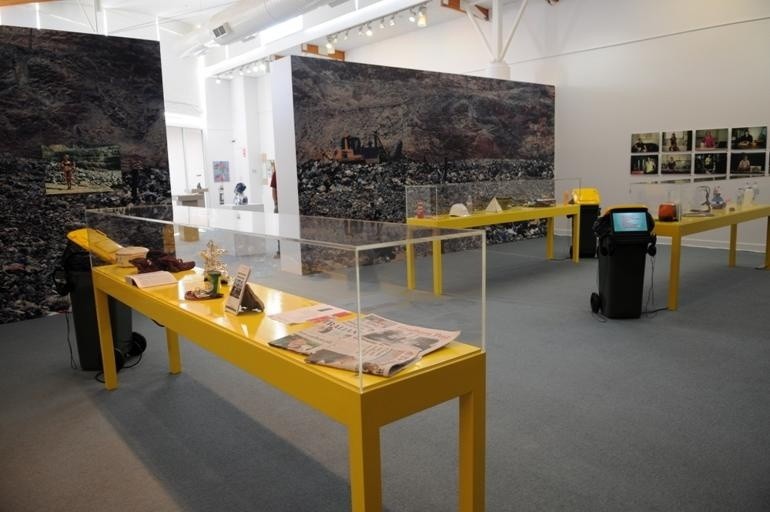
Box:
[669,134,677,152]
[737,155,750,170]
[642,156,656,174]
[61,154,76,191]
[739,130,753,142]
[704,130,715,147]
[634,138,646,152]
[705,154,712,169]
[666,157,676,169]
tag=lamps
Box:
[216,58,266,85]
[326,1,431,47]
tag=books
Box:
[124,270,179,289]
[683,211,715,217]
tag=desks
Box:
[408,205,580,296]
[92,263,486,511]
[651,201,770,311]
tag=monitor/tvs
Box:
[612,212,649,233]
